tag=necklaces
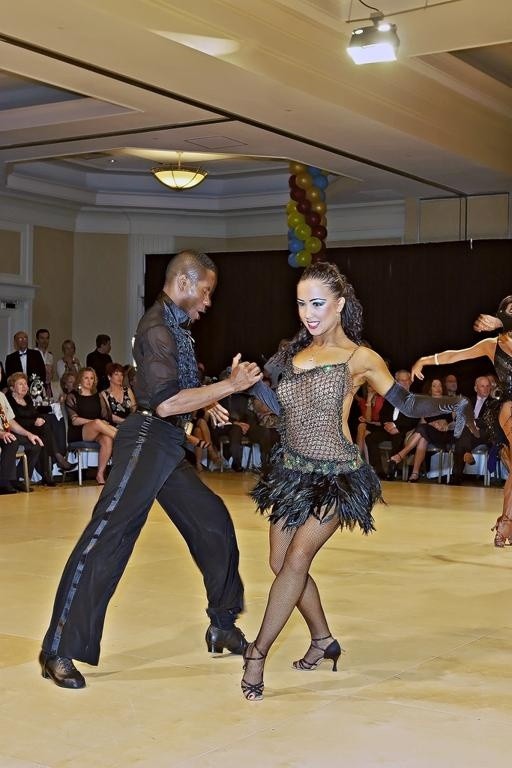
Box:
[306,339,349,363]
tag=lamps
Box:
[149,149,209,190]
[347,11,400,64]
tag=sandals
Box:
[196,440,211,449]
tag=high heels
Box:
[41,476,55,486]
[491,514,512,547]
[293,634,341,671]
[58,463,78,474]
[241,641,267,701]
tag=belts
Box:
[136,407,185,428]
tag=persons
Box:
[2,329,136,495]
[38,247,263,686]
[193,340,290,474]
[473,314,503,333]
[347,362,497,486]
[221,260,485,702]
[410,297,511,549]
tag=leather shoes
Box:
[40,652,85,688]
[386,453,420,483]
[1,473,33,494]
[206,626,250,654]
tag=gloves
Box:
[383,381,479,439]
[227,366,280,417]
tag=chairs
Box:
[16,395,500,491]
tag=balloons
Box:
[284,157,329,269]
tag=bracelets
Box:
[435,354,439,365]
